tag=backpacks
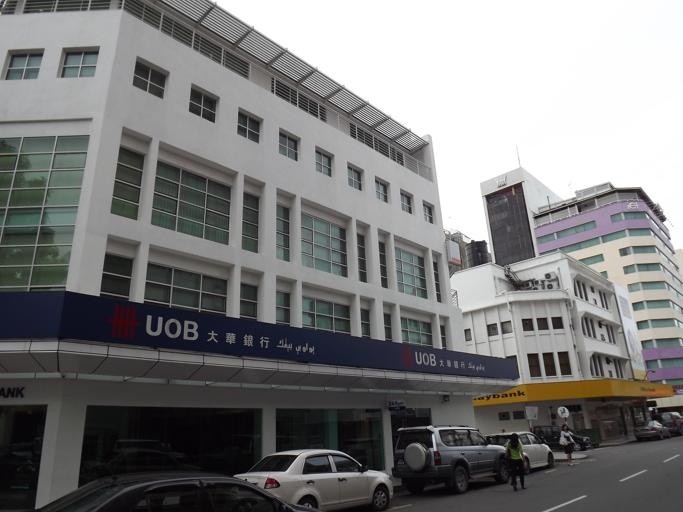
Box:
[560,438,567,446]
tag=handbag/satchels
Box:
[505,449,511,458]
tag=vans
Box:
[486,431,554,474]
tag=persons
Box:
[559,424,575,466]
[505,433,527,491]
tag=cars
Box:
[634,412,683,441]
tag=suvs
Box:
[393,424,512,493]
[533,424,592,451]
